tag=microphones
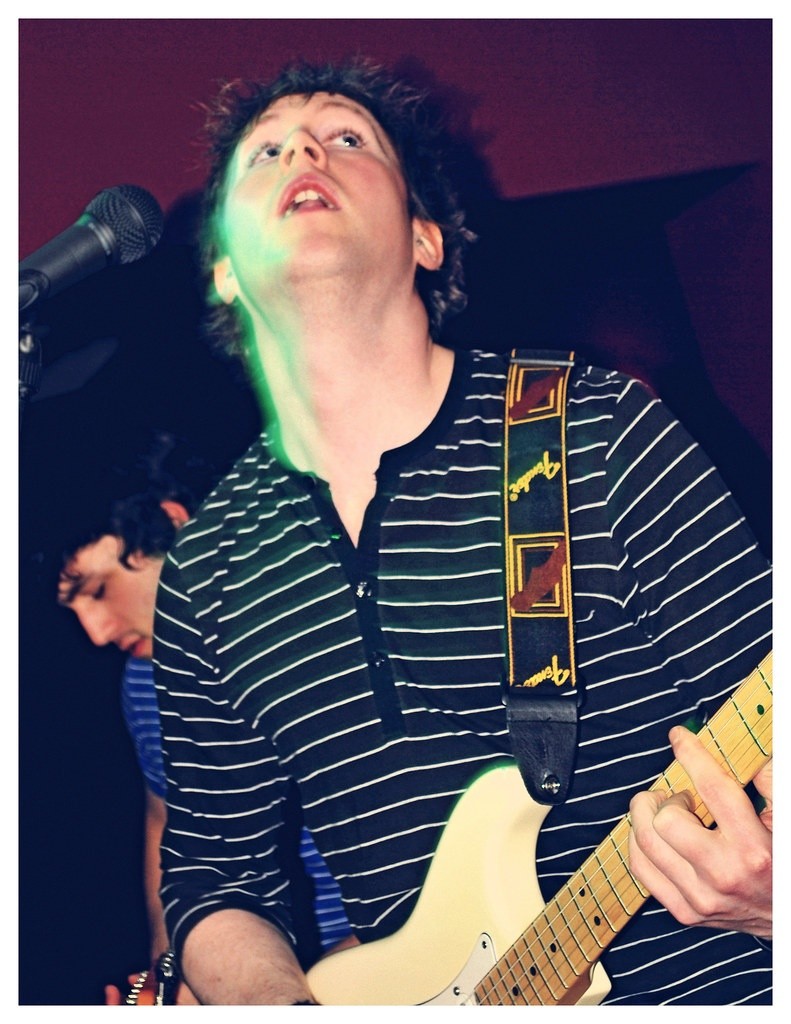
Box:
[19,184,163,309]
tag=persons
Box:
[155,51,772,1005]
[45,401,359,1005]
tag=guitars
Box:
[299,647,773,1005]
